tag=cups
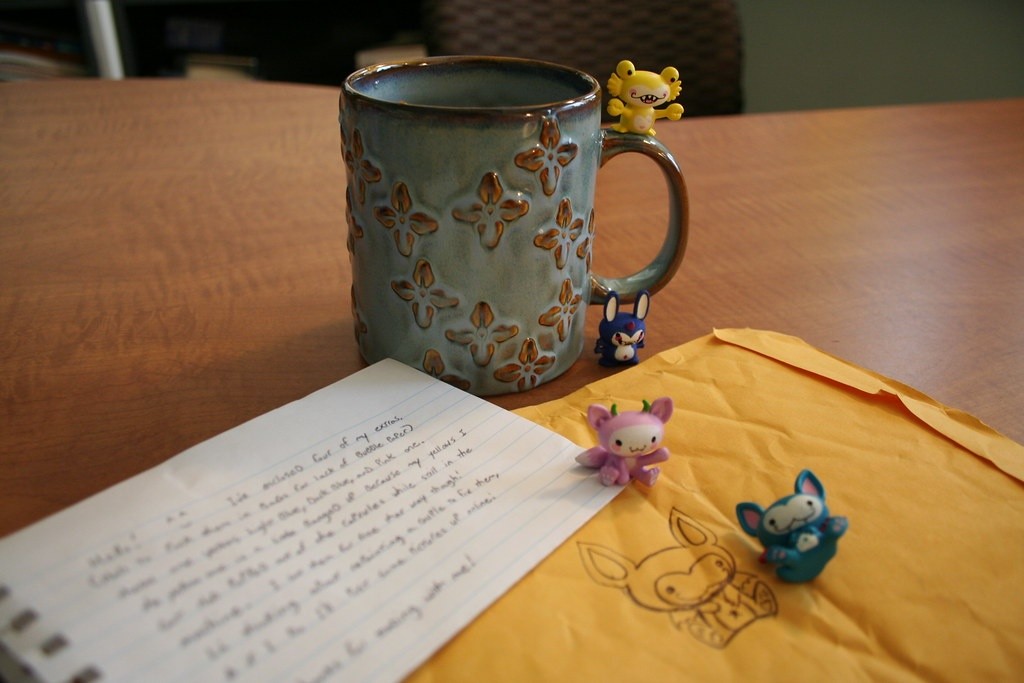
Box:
[339,58,689,394]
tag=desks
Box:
[2,71,1022,683]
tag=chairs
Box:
[427,0,744,126]
